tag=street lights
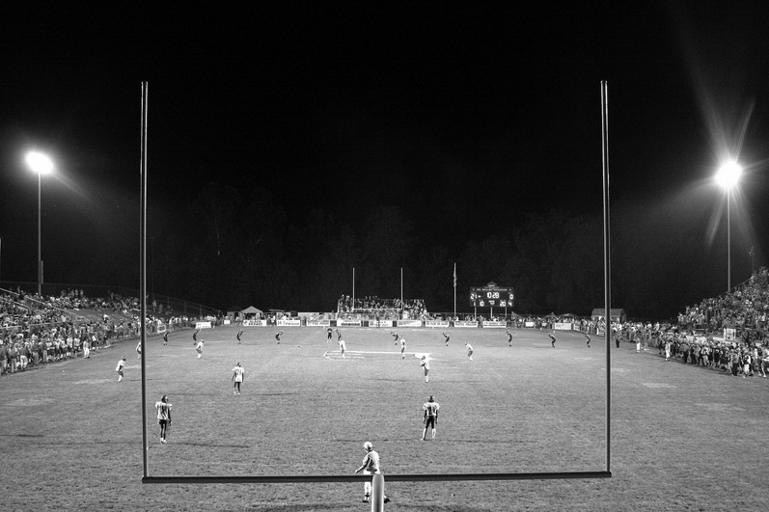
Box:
[711,159,744,296]
[24,150,54,297]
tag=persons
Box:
[1,262,769,398]
[352,441,389,503]
[154,394,172,444]
[421,394,440,441]
